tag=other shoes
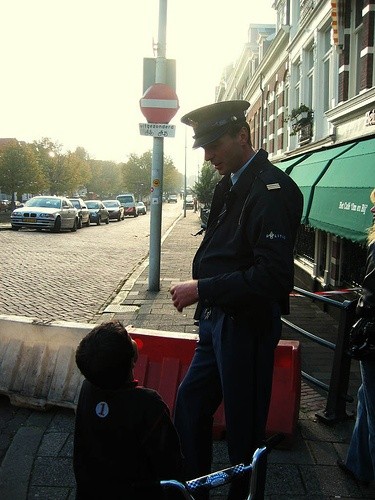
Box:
[337,462,370,489]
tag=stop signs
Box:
[139,83,179,124]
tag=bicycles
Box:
[145,432,286,500]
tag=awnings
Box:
[273,139,375,241]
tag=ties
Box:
[204,175,233,242]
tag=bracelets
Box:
[196,281,198,298]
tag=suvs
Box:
[116,194,139,217]
[168,195,177,203]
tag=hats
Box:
[183,101,250,148]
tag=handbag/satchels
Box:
[347,317,375,361]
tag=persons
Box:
[336,188,375,488]
[166,99,304,500]
[73,322,184,500]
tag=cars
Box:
[11,196,79,233]
[102,200,124,221]
[137,202,146,215]
[83,200,109,225]
[68,198,90,229]
[183,198,195,209]
[0,200,24,212]
